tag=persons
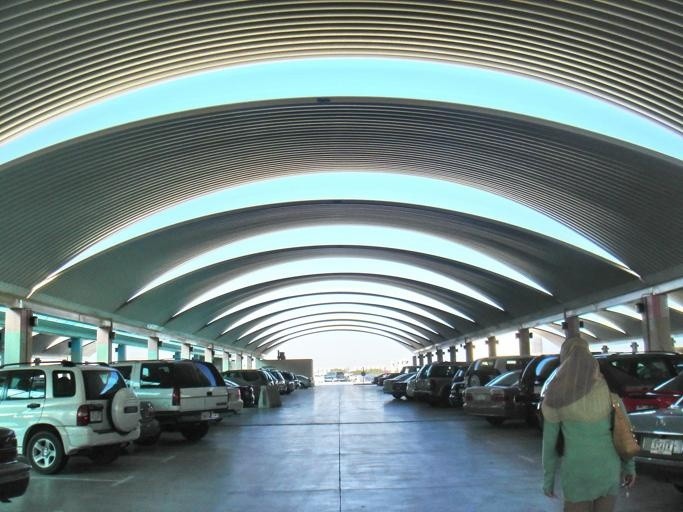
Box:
[540,335,637,511]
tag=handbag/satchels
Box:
[609,392,641,461]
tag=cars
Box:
[371,350,683,492]
[0,426,30,505]
[221,368,314,416]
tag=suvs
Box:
[0,358,142,476]
[324,372,345,382]
[108,359,230,447]
